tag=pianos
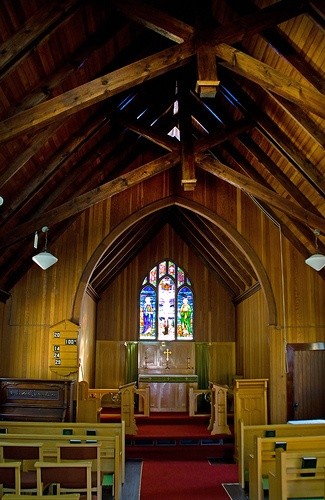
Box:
[0,377,76,424]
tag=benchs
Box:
[267,448,325,500]
[237,419,325,489]
[0,434,119,500]
[77,380,103,422]
[0,420,125,486]
[248,435,325,500]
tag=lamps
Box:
[304,253,325,272]
[32,250,59,270]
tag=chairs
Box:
[1,492,81,500]
[32,461,92,500]
[0,461,21,495]
[56,441,103,500]
[0,442,50,493]
